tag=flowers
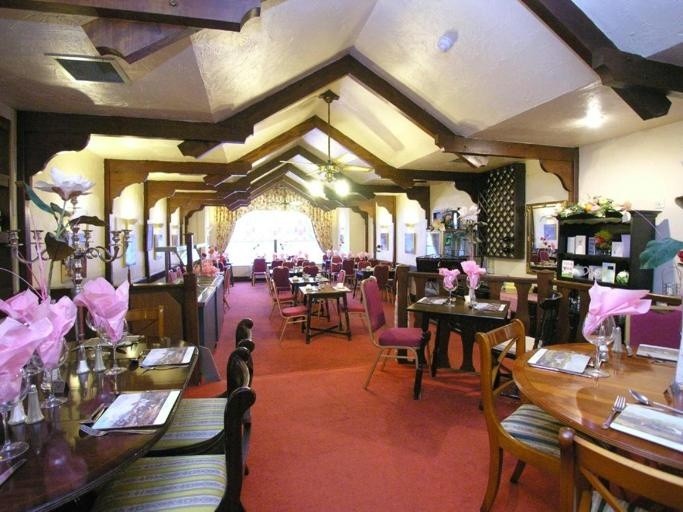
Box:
[21,167,109,298]
[556,196,614,218]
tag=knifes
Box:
[624,339,633,358]
[0,459,28,484]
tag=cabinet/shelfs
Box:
[555,211,662,290]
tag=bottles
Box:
[25,384,44,424]
[93,343,106,373]
[7,401,27,426]
[75,343,90,374]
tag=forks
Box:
[79,424,157,436]
[601,394,625,429]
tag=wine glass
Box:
[443,277,458,307]
[85,307,127,376]
[582,311,617,377]
[26,337,68,409]
[466,276,482,304]
[0,370,30,462]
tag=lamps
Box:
[320,165,340,184]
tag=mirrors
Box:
[526,200,568,274]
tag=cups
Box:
[337,282,343,289]
[303,274,308,280]
[306,284,312,290]
[293,276,297,280]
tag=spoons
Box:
[129,351,143,361]
[79,402,104,424]
[628,388,683,416]
[647,353,676,367]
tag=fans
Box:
[279,89,376,184]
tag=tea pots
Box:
[575,263,589,277]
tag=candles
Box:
[114,214,117,230]
[85,210,89,230]
